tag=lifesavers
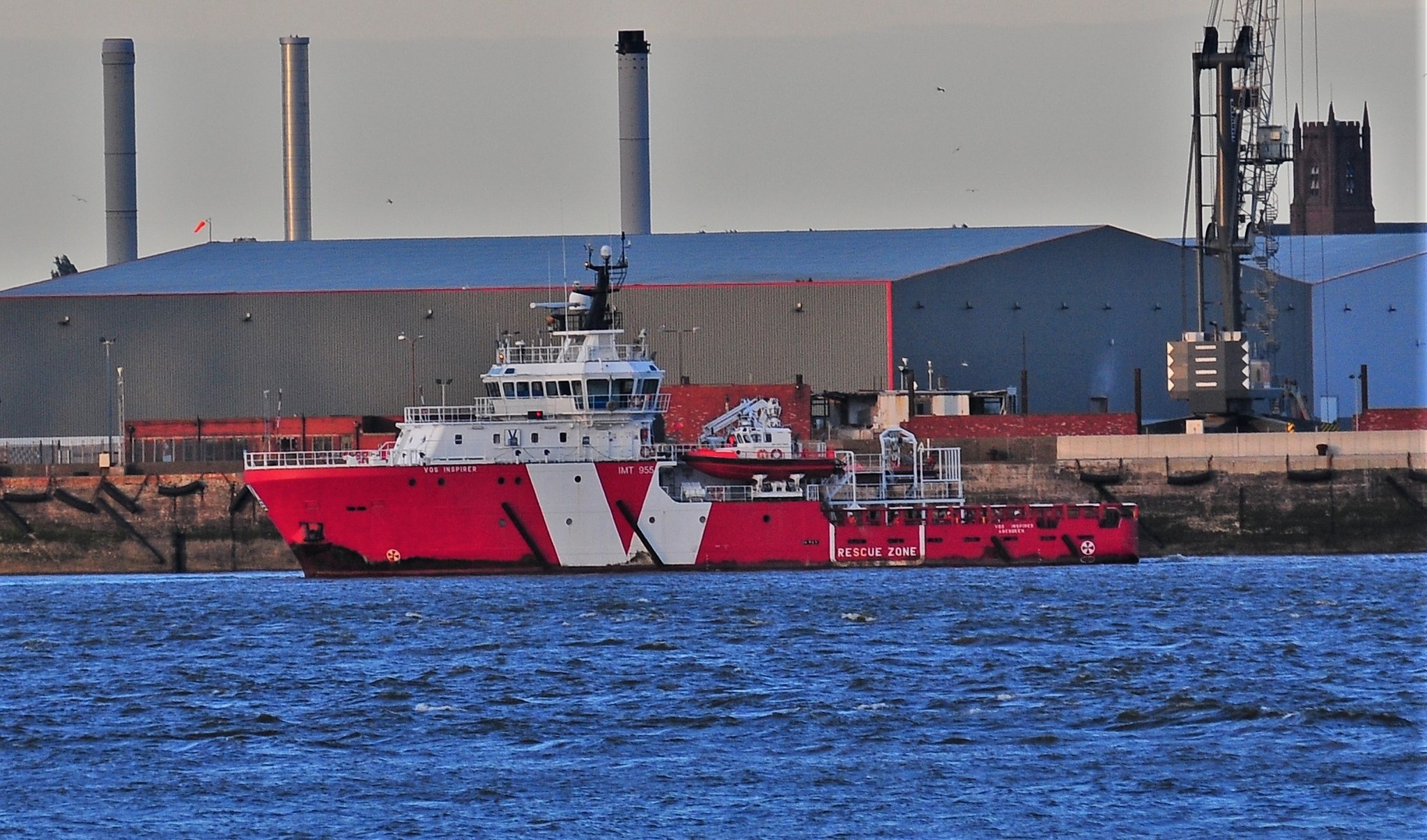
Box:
[639,445,652,458]
[770,448,782,459]
[757,450,768,459]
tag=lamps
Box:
[1014,302,1021,310]
[426,309,433,318]
[1102,303,1111,310]
[1286,303,1295,310]
[966,300,973,309]
[797,303,805,313]
[1154,303,1162,311]
[1343,303,1351,311]
[244,312,252,321]
[1060,302,1069,309]
[917,301,925,309]
[1388,304,1396,311]
[61,315,70,325]
[1244,301,1252,310]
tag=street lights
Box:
[99,336,117,468]
[658,326,701,384]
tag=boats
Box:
[678,396,847,484]
[239,233,1140,581]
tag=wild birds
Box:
[385,198,392,204]
